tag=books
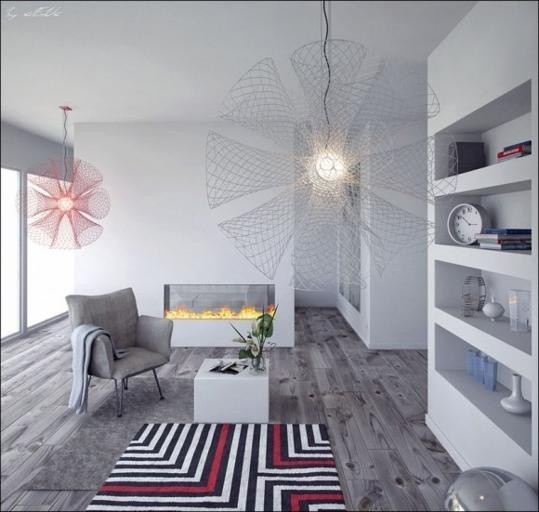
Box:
[497,140,532,163]
[474,227,532,251]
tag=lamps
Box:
[204,1,460,294]
[13,107,112,252]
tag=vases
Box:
[507,288,534,334]
[499,373,533,417]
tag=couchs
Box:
[65,287,174,418]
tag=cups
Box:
[483,361,498,392]
[508,290,531,332]
[467,349,481,376]
[473,356,488,384]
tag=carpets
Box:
[85,422,348,512]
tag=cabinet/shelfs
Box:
[163,282,277,322]
[424,58,538,512]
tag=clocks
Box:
[446,201,493,247]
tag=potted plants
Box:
[227,302,282,376]
